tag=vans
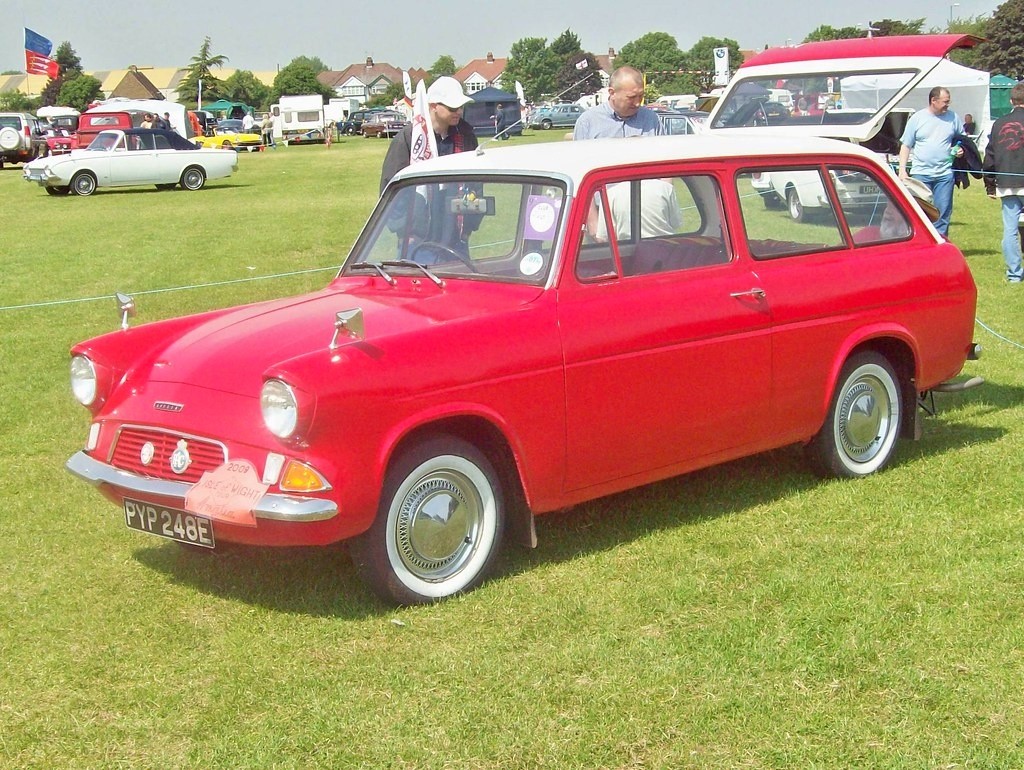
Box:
[655,95,699,108]
[711,88,794,112]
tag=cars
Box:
[645,96,787,180]
[527,104,586,130]
[39,109,413,155]
[22,128,239,197]
[61,34,985,607]
[769,107,914,222]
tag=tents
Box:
[728,85,775,119]
[460,86,523,136]
[841,59,1020,163]
[81,100,256,141]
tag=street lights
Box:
[950,3,961,20]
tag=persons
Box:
[378,76,483,268]
[572,66,682,243]
[897,87,969,238]
[260,114,272,147]
[961,114,975,135]
[983,84,1024,283]
[268,112,276,143]
[139,111,177,149]
[242,111,254,135]
[490,104,509,141]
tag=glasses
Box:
[438,103,458,112]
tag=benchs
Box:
[627,238,727,274]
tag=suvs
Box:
[0,112,49,168]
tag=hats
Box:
[427,76,475,108]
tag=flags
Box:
[402,72,417,123]
[24,28,52,56]
[404,78,446,203]
[25,50,59,80]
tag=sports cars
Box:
[188,127,262,152]
